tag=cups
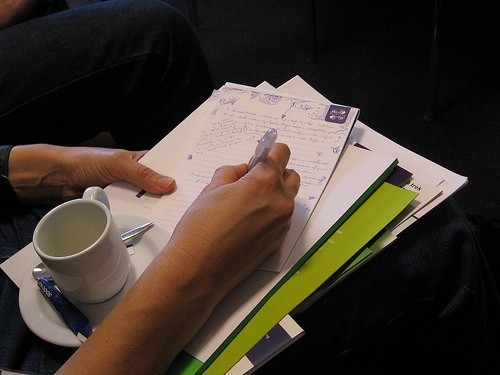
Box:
[32,187,130,303]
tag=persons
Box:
[0,143,300,375]
[0,0,213,151]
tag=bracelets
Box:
[0,145,31,217]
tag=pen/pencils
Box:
[247,127,276,175]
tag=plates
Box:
[18,214,171,348]
